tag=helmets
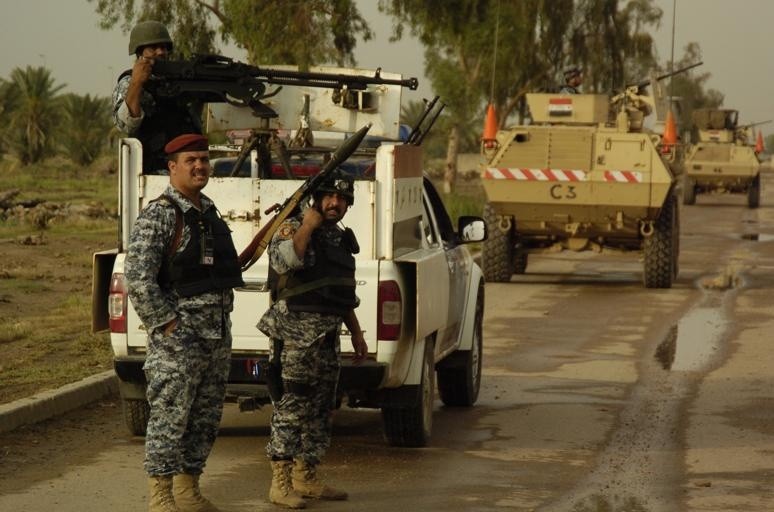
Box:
[128,21,174,57]
[312,169,356,205]
[564,65,580,80]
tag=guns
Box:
[365,94,441,178]
[410,104,446,148]
[143,53,420,109]
[625,62,703,95]
[737,119,773,130]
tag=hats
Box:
[165,133,210,154]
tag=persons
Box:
[124,134,246,512]
[256,168,368,508]
[112,21,203,174]
[559,64,584,94]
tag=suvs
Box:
[90,63,486,447]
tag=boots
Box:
[268,460,308,508]
[173,474,222,512]
[291,470,348,501]
[147,474,183,512]
